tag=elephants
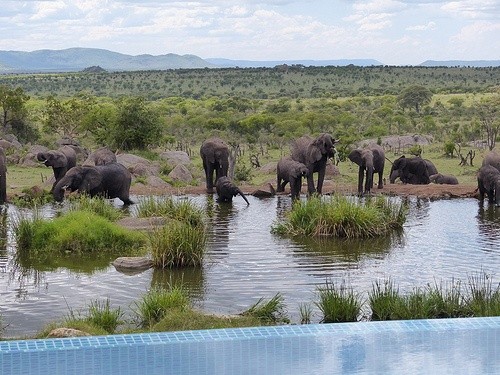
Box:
[0,130,500,215]
